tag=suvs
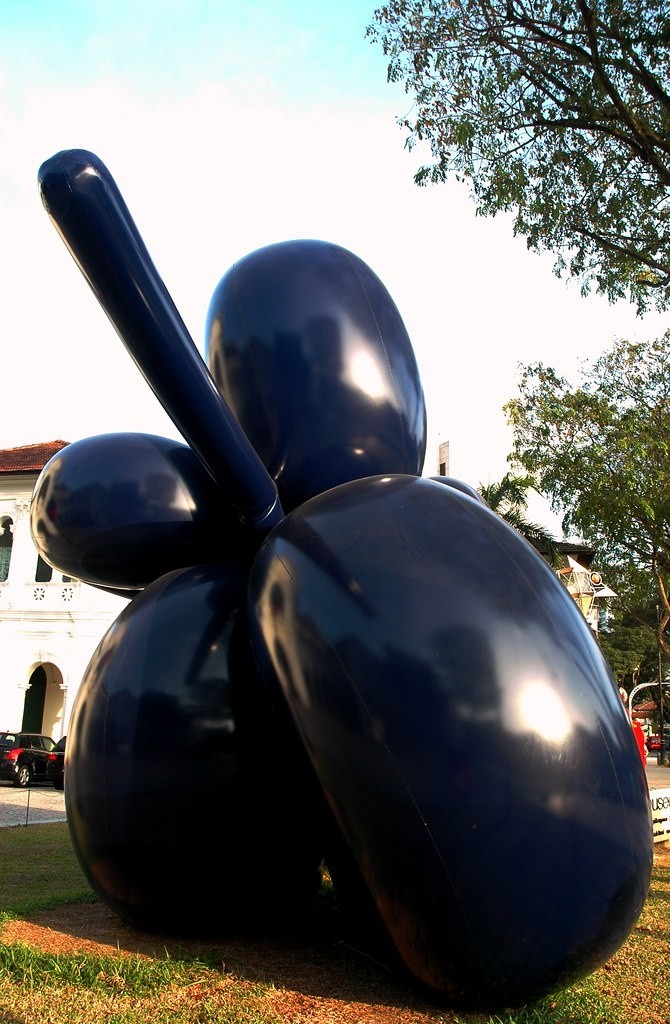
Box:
[0,730,56,788]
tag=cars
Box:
[646,736,660,751]
[47,736,66,790]
